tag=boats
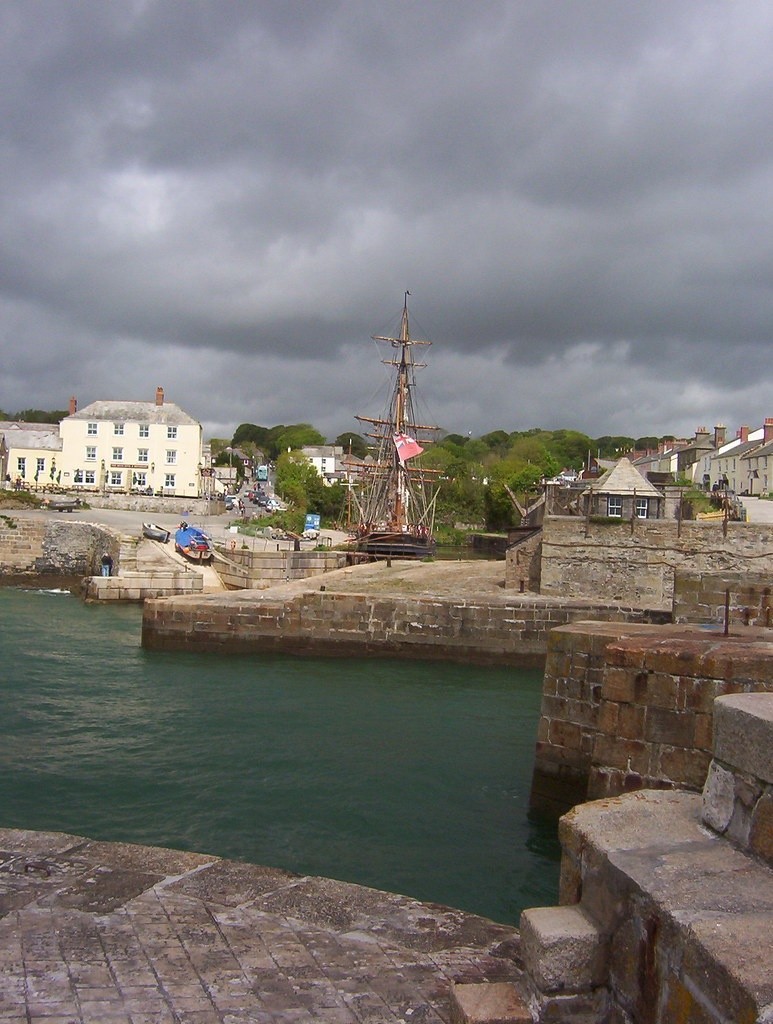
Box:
[174,525,215,564]
[49,498,80,509]
[142,522,171,544]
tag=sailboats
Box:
[341,290,449,562]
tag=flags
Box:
[392,431,424,461]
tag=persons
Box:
[138,483,144,492]
[6,473,12,488]
[102,552,113,577]
[203,466,263,517]
[146,485,152,495]
[16,475,22,491]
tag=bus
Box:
[257,466,268,480]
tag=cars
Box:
[224,489,288,512]
